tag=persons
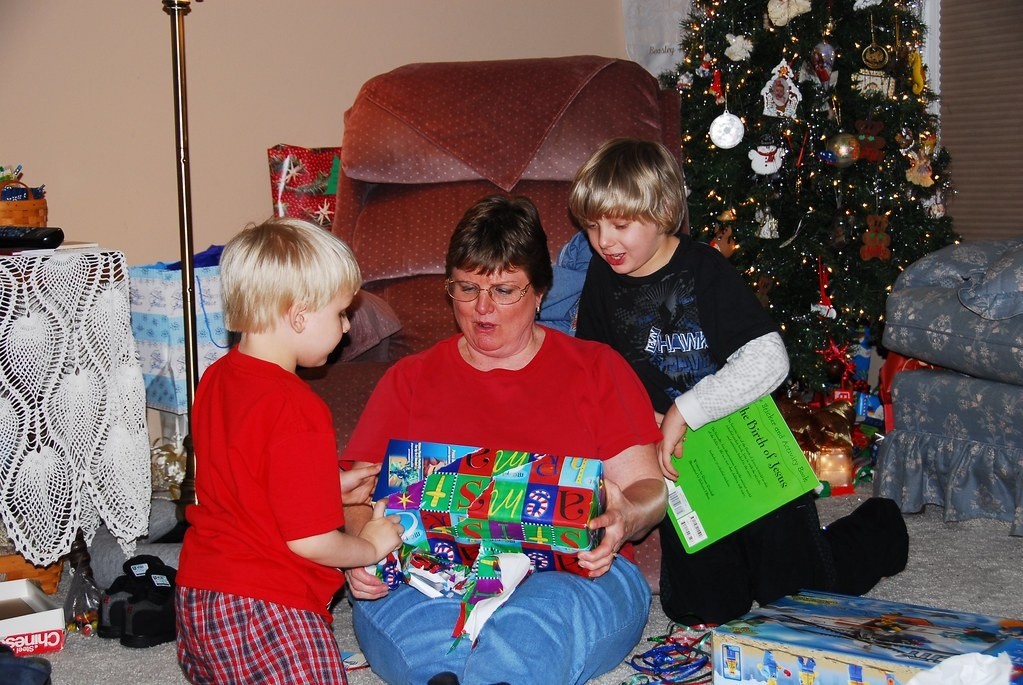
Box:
[344,193,668,685]
[569,136,909,624]
[174,217,405,685]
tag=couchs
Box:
[297,53,688,593]
[873,285,1023,534]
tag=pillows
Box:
[958,237,1023,320]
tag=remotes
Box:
[0,225,64,249]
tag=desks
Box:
[0,249,153,595]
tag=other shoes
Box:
[97,554,165,638]
[120,565,177,649]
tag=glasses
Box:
[445,275,532,305]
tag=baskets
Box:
[0,180,48,228]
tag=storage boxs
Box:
[827,325,884,427]
[0,579,66,657]
[370,435,606,579]
[710,589,1023,684]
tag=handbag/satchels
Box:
[125,262,233,415]
[268,142,343,232]
